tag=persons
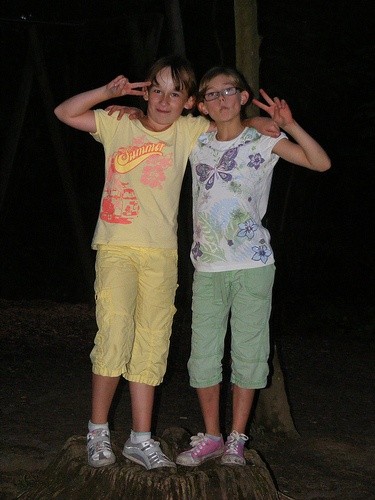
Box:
[170,66,332,468]
[54,61,281,474]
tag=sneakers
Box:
[86,429,116,468]
[221,430,249,467]
[121,437,177,472]
[174,431,225,467]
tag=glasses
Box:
[201,87,240,102]
